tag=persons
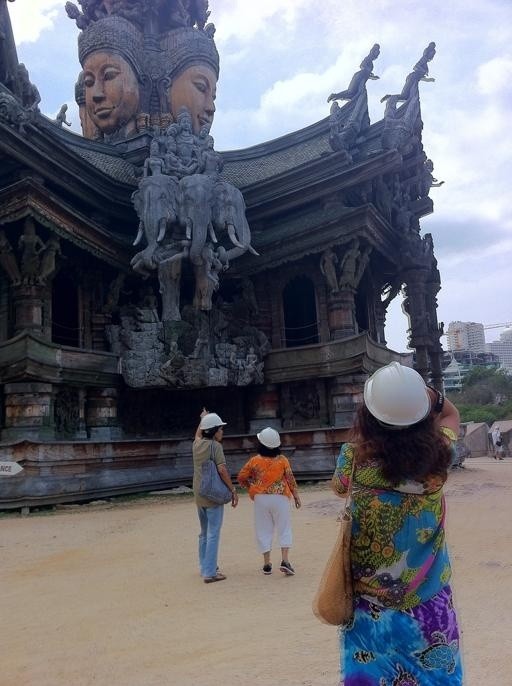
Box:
[380,38,436,103]
[77,18,147,135]
[192,332,207,358]
[326,42,379,103]
[142,109,221,178]
[316,239,374,293]
[68,75,102,142]
[192,406,238,583]
[332,360,464,686]
[229,344,240,369]
[236,426,301,576]
[159,28,221,137]
[246,347,258,368]
[491,426,506,460]
[171,333,179,354]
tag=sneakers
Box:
[493,454,504,460]
[279,560,295,575]
[263,562,273,574]
[200,565,226,583]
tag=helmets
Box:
[257,426,281,449]
[200,413,228,430]
[364,361,431,426]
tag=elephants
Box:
[130,173,261,271]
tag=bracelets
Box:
[231,487,236,492]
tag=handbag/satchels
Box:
[496,441,502,446]
[312,506,353,625]
[199,460,232,505]
